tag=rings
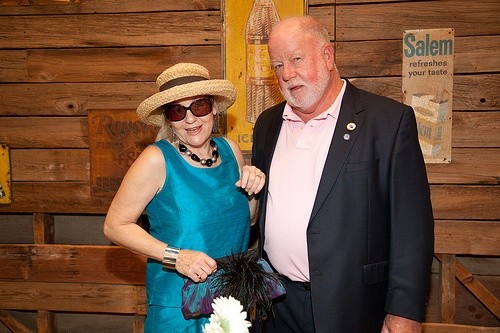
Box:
[256,175,261,179]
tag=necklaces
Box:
[178,138,219,166]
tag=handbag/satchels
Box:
[181,249,287,320]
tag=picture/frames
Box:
[221,0,308,156]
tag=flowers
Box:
[199,294,251,333]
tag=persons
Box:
[248,16,434,333]
[104,63,266,333]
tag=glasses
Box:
[164,99,213,122]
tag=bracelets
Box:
[162,244,182,270]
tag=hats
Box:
[135,62,237,128]
